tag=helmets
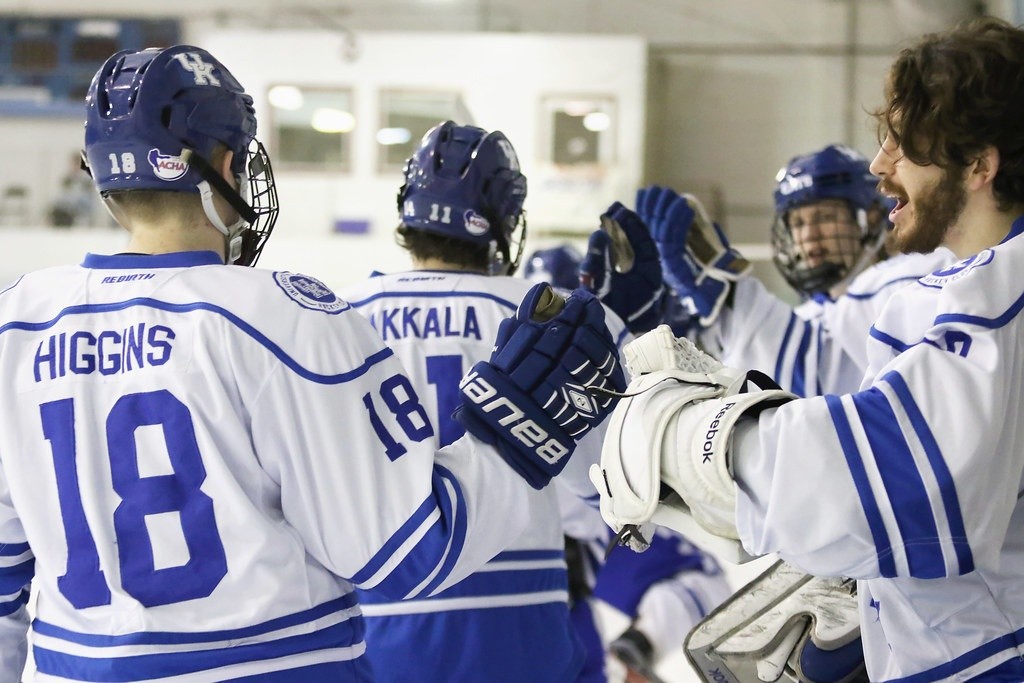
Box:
[523,247,583,289]
[770,145,898,304]
[81,44,279,267]
[399,121,527,276]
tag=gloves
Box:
[450,281,627,490]
[578,201,672,334]
[635,184,755,325]
[589,325,809,551]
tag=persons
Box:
[0,18,1024,683]
